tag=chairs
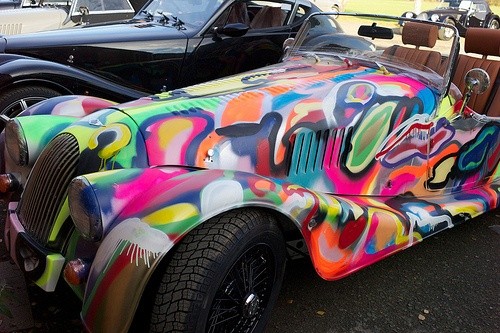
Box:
[379,19,441,85]
[441,27,500,120]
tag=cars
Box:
[0,1,345,135]
[1,11,499,333]
[397,0,500,41]
